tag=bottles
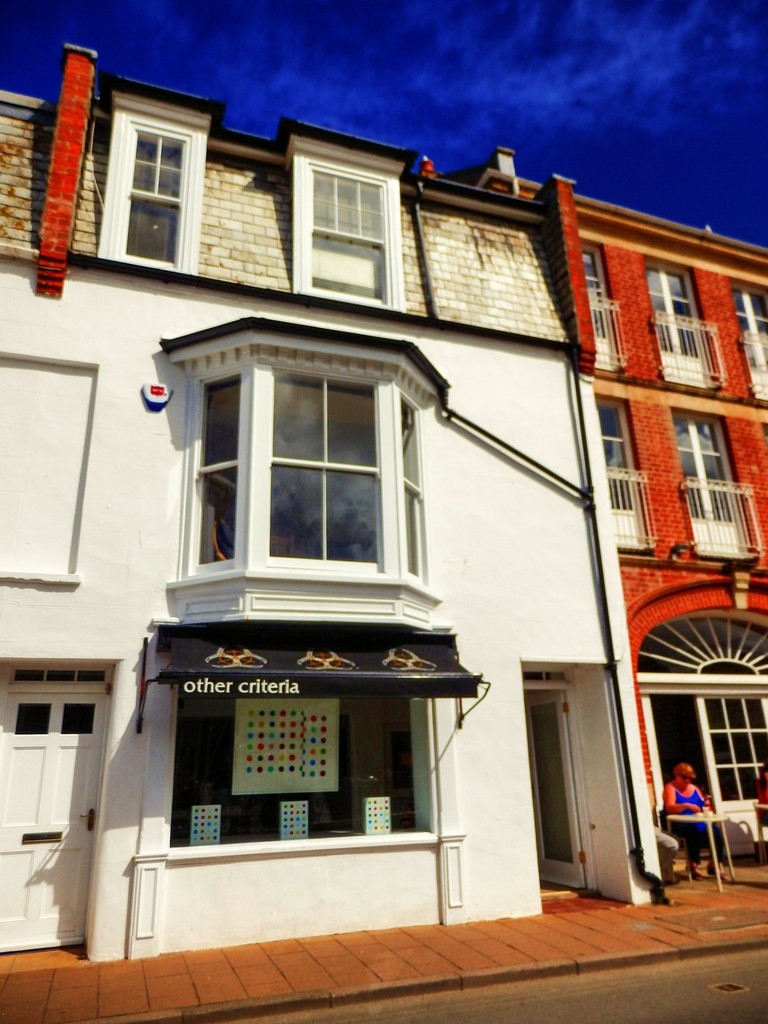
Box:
[707,795,713,816]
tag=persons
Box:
[663,763,730,882]
[756,760,768,826]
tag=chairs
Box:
[659,810,724,882]
[753,802,768,866]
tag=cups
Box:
[702,807,709,817]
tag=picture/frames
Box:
[383,722,414,798]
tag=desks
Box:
[666,814,736,894]
